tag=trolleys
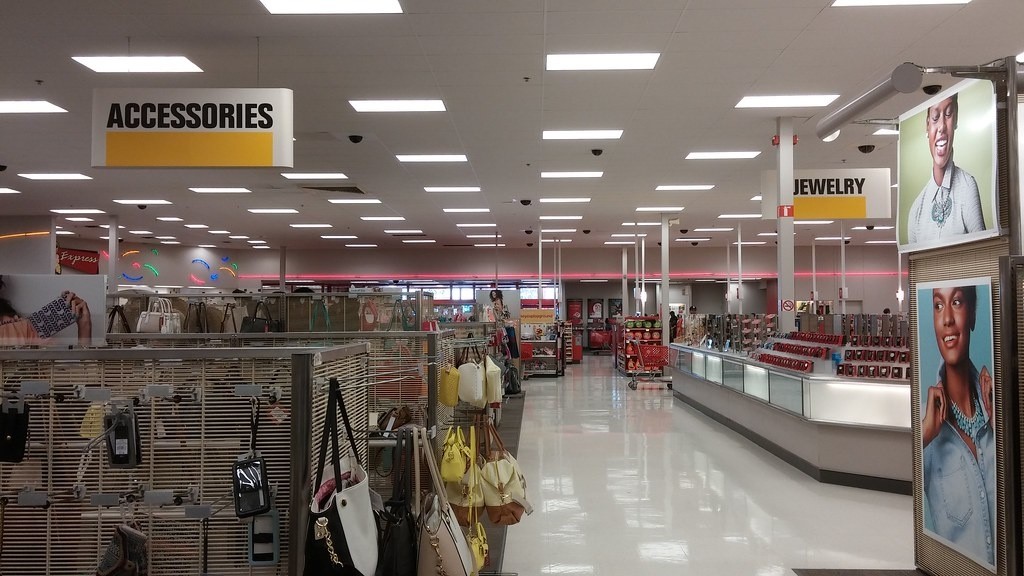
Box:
[628,341,673,390]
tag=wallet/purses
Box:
[96,499,150,576]
[247,508,280,566]
[83,410,143,469]
[231,398,272,519]
[0,390,30,463]
[26,293,79,340]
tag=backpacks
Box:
[502,360,522,394]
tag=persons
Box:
[669,311,683,341]
[921,286,996,566]
[689,306,696,314]
[0,275,91,349]
[908,92,986,243]
[489,290,503,313]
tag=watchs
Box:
[838,337,911,378]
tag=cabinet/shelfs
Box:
[521,321,574,378]
[614,316,665,377]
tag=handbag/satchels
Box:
[374,334,534,576]
[301,377,380,576]
[106,297,414,333]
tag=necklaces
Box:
[935,169,955,221]
[945,393,985,442]
